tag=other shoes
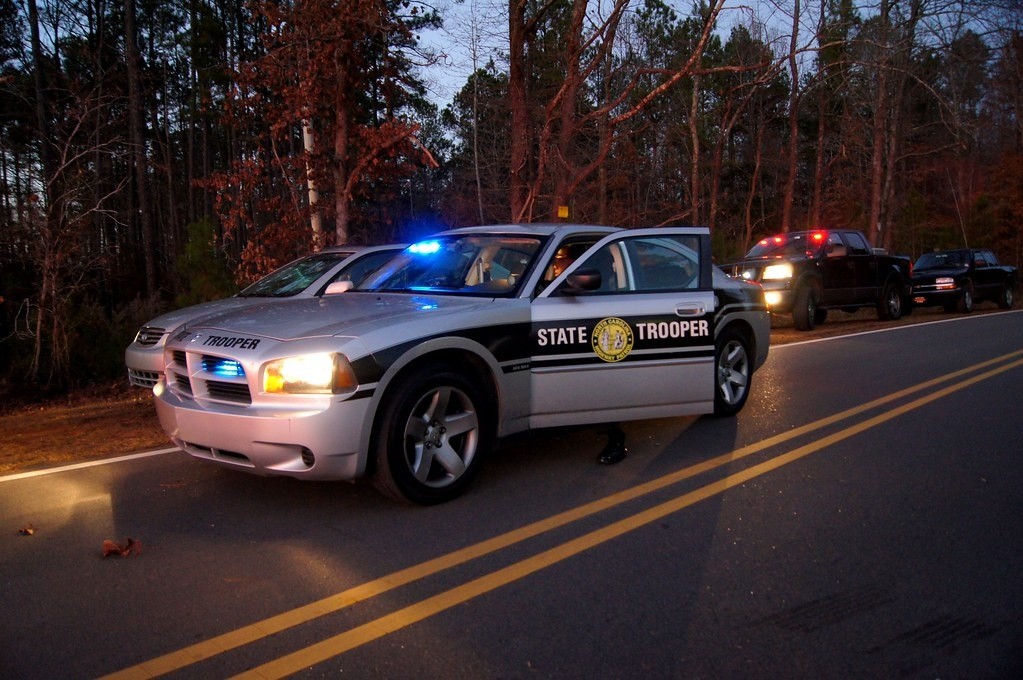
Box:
[598,434,627,464]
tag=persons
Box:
[530,246,631,466]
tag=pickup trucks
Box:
[912,248,1019,314]
[715,227,914,332]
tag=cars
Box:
[151,223,773,508]
[122,241,558,395]
